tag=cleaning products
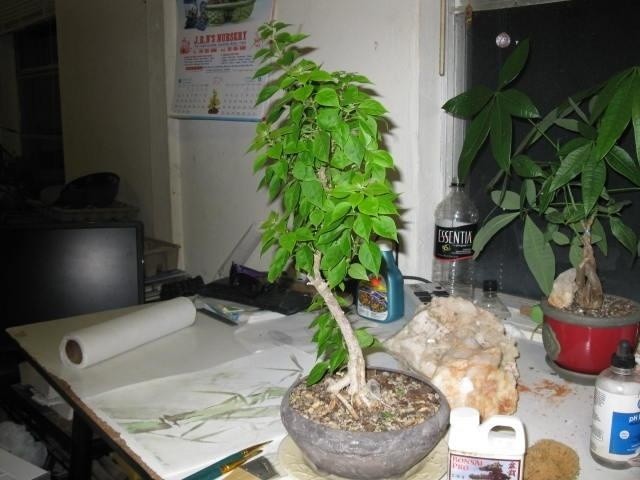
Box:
[355,238,405,325]
[446,406,525,480]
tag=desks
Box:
[6,292,640,480]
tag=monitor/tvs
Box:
[0,220,145,331]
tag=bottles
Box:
[588,340,640,470]
[475,280,510,322]
[431,176,479,302]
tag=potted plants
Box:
[441,38,640,377]
[238,15,452,479]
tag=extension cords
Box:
[403,280,451,319]
